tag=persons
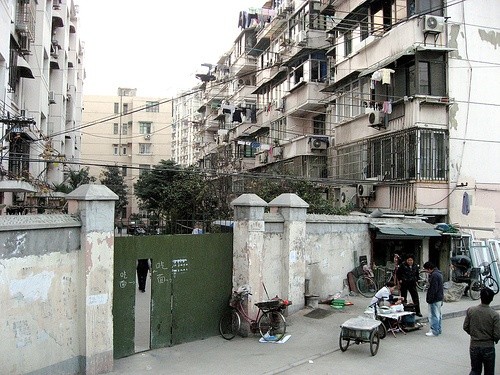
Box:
[396,254,423,317]
[393,250,403,288]
[463,288,500,375]
[451,255,472,276]
[368,282,403,332]
[423,262,444,336]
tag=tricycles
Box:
[339,296,388,356]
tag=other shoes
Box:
[425,330,435,336]
[416,313,422,316]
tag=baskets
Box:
[478,261,490,276]
[230,291,244,307]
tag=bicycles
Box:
[469,260,499,300]
[353,260,378,298]
[218,287,287,343]
[416,265,431,291]
